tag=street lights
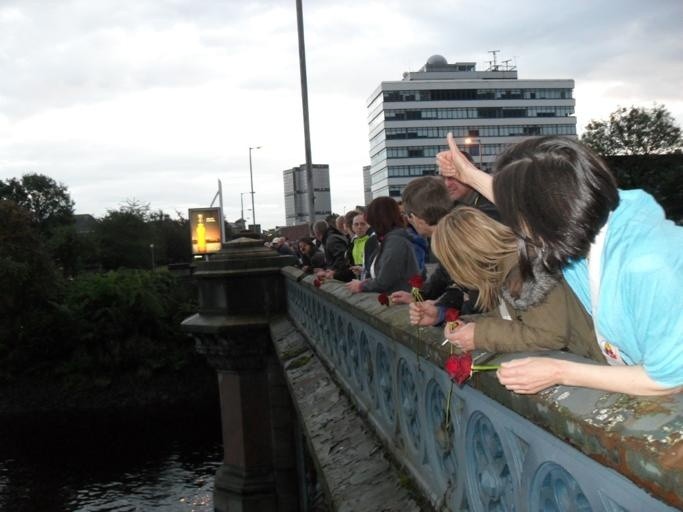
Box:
[240,146,262,234]
[464,137,482,169]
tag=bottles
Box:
[196,214,207,254]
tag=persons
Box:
[388,150,606,365]
[263,196,429,293]
[434,129,683,397]
